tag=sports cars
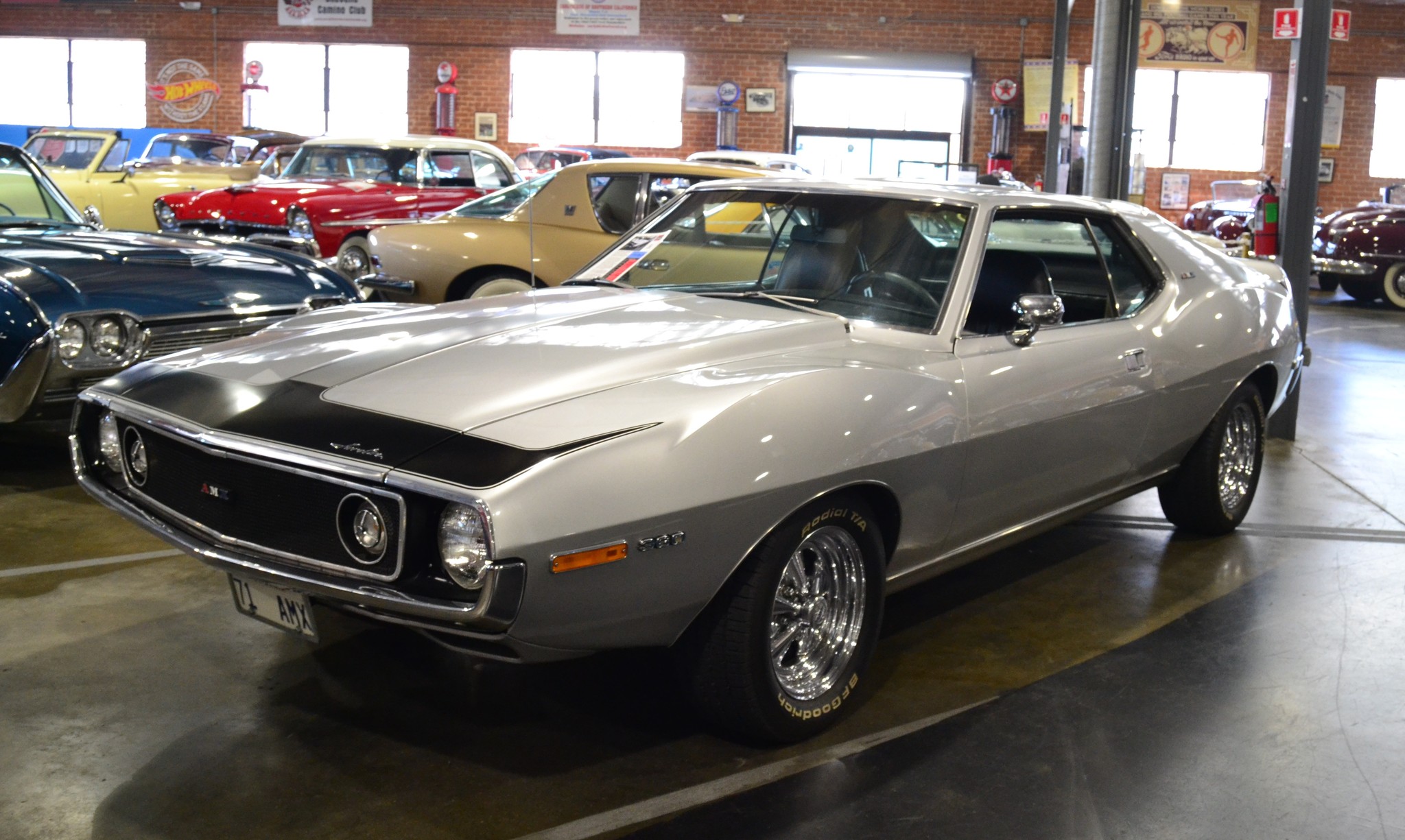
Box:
[63,165,1308,749]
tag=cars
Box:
[1311,184,1405,312]
[0,125,817,307]
[0,140,363,467]
[1177,178,1261,246]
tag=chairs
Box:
[775,225,871,298]
[963,249,1054,335]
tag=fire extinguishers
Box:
[1255,175,1280,256]
[1034,174,1043,193]
